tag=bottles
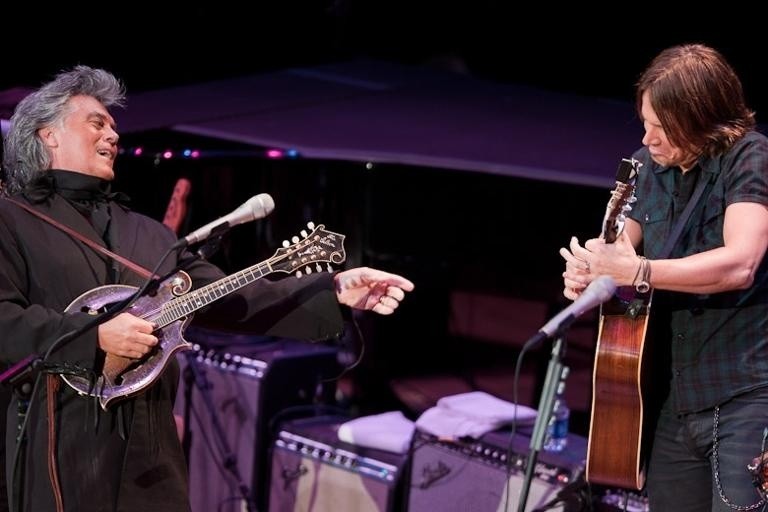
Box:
[543,394,570,453]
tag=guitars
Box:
[586,158,655,492]
[57,220,346,411]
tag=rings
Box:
[585,262,590,274]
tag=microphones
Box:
[526,272,619,351]
[170,191,277,252]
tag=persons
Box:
[560,45,768,512]
[0,65,416,511]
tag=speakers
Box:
[173,334,649,512]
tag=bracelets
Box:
[631,255,652,295]
